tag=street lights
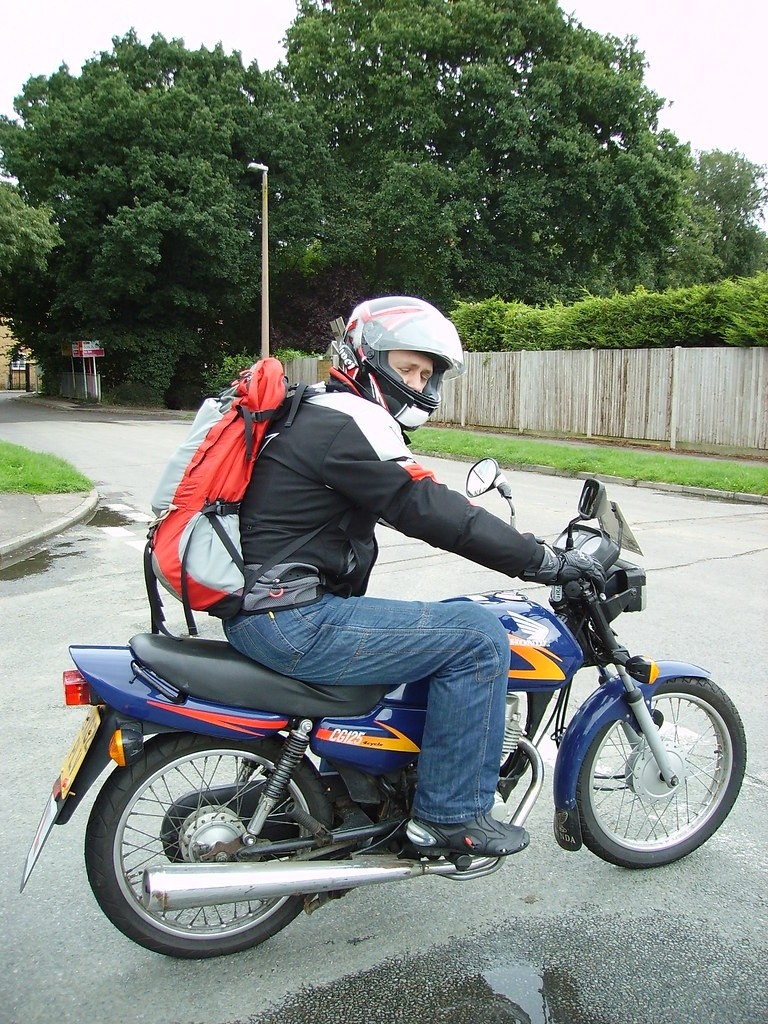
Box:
[246,162,272,362]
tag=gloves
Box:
[528,544,607,592]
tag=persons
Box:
[220,298,607,855]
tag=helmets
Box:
[329,295,467,432]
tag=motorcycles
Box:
[18,457,748,958]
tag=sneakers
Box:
[404,816,530,858]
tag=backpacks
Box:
[142,356,365,643]
